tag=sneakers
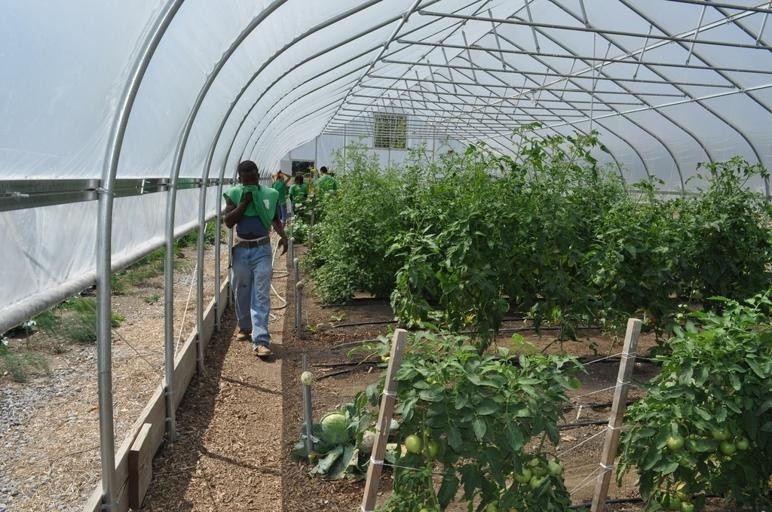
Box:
[236,329,250,341]
[251,346,275,357]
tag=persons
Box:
[314,166,338,192]
[271,169,290,227]
[222,160,289,357]
[289,174,308,224]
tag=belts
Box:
[237,237,270,248]
